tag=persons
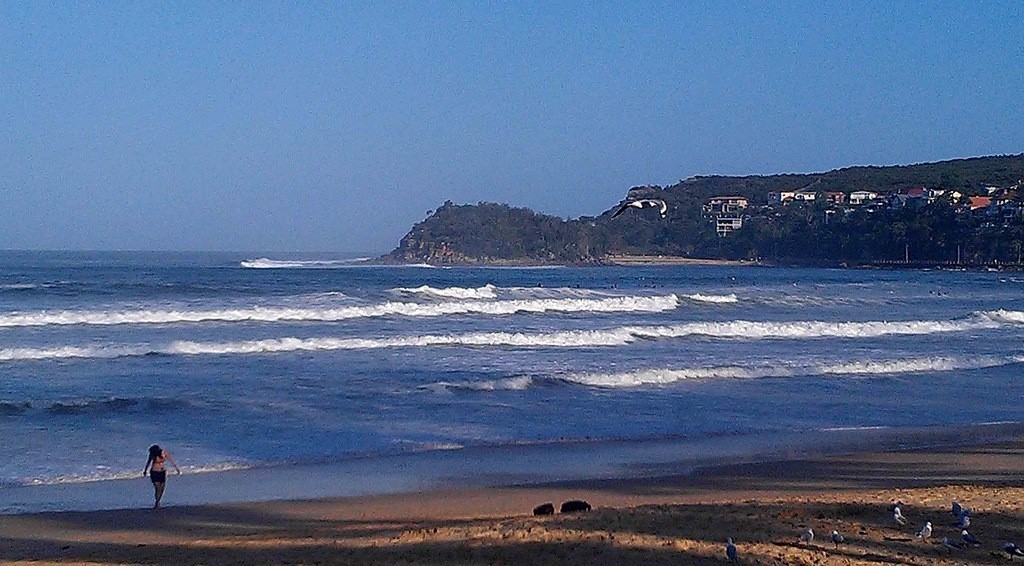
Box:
[143,444,180,510]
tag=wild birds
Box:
[1002,540,1024,563]
[941,501,983,552]
[726,537,739,565]
[799,528,814,546]
[914,520,933,545]
[888,504,908,525]
[830,530,844,547]
